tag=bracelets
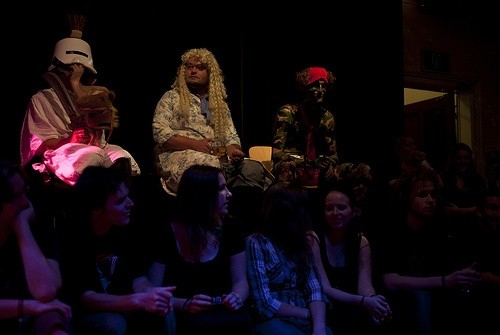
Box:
[304,309,308,318]
[369,295,375,297]
[181,298,190,310]
[360,296,365,305]
[18,299,23,320]
[441,274,448,289]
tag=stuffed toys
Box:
[76,89,118,128]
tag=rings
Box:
[231,292,235,295]
[235,294,238,298]
[239,297,243,303]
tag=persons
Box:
[276,67,359,189]
[152,46,268,199]
[0,140,500,335]
[20,37,141,185]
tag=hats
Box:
[296,67,336,88]
[52,38,97,75]
